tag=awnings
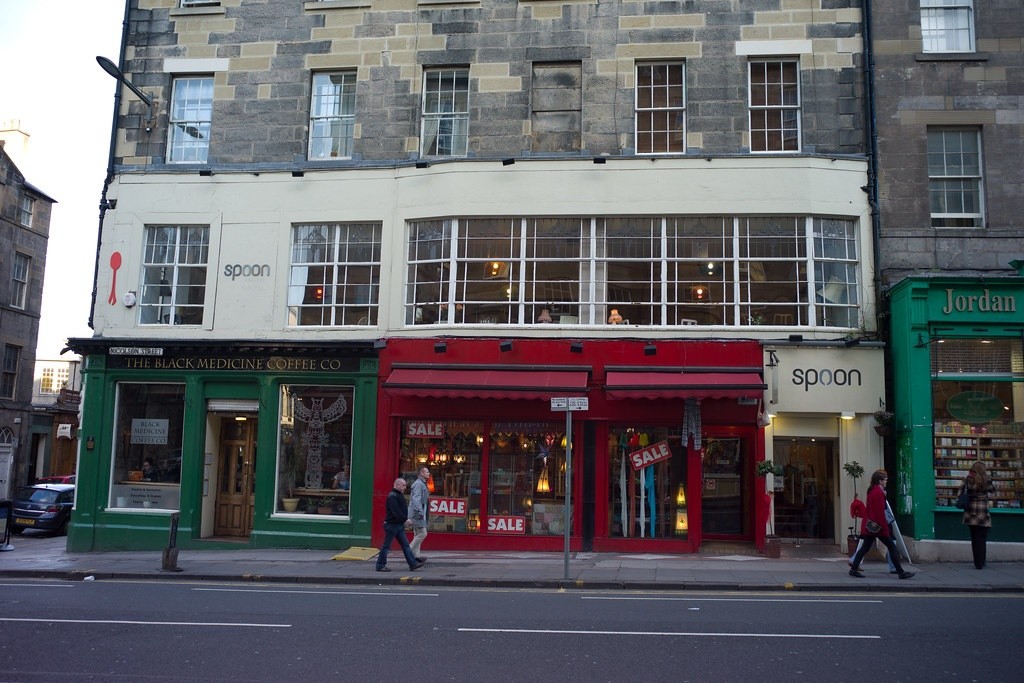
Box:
[603,371,768,399]
[381,368,588,402]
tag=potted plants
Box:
[756,460,781,558]
[317,496,336,514]
[303,497,317,514]
[873,411,894,438]
[281,472,299,512]
[843,460,864,558]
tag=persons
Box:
[308,464,338,489]
[141,460,160,482]
[848,471,923,579]
[957,462,996,569]
[376,478,424,572]
[407,467,431,562]
[333,462,350,490]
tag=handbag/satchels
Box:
[866,519,881,534]
[956,476,968,509]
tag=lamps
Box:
[374,339,387,349]
[434,342,448,353]
[841,411,855,419]
[499,341,513,352]
[643,345,656,355]
[608,310,622,324]
[571,343,584,352]
[818,276,847,326]
[538,310,552,322]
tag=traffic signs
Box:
[550,397,568,411]
[569,397,589,411]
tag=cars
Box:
[399,470,446,496]
[9,475,75,535]
[298,442,350,486]
[490,471,531,496]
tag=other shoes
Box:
[891,569,900,573]
[415,556,427,562]
[376,565,392,572]
[849,569,866,578]
[898,570,917,579]
[410,563,423,571]
[848,560,864,572]
